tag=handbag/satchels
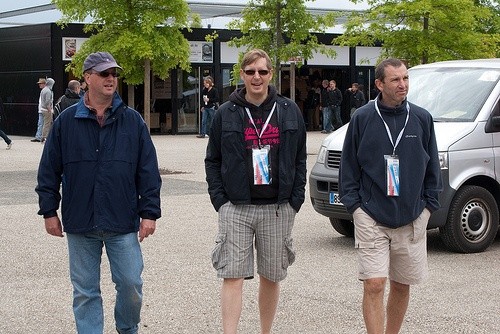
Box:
[215,102,219,110]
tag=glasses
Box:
[241,69,271,76]
[89,71,120,77]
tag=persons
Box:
[338,58,444,334]
[205,49,307,334]
[307,78,366,133]
[31,78,87,142]
[35,51,163,334]
[0,130,13,150]
[196,76,218,138]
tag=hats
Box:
[322,80,329,83]
[83,51,123,72]
[38,78,46,84]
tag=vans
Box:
[308,56,500,254]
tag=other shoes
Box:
[196,134,205,138]
[6,141,13,149]
[31,138,42,141]
[321,130,329,133]
